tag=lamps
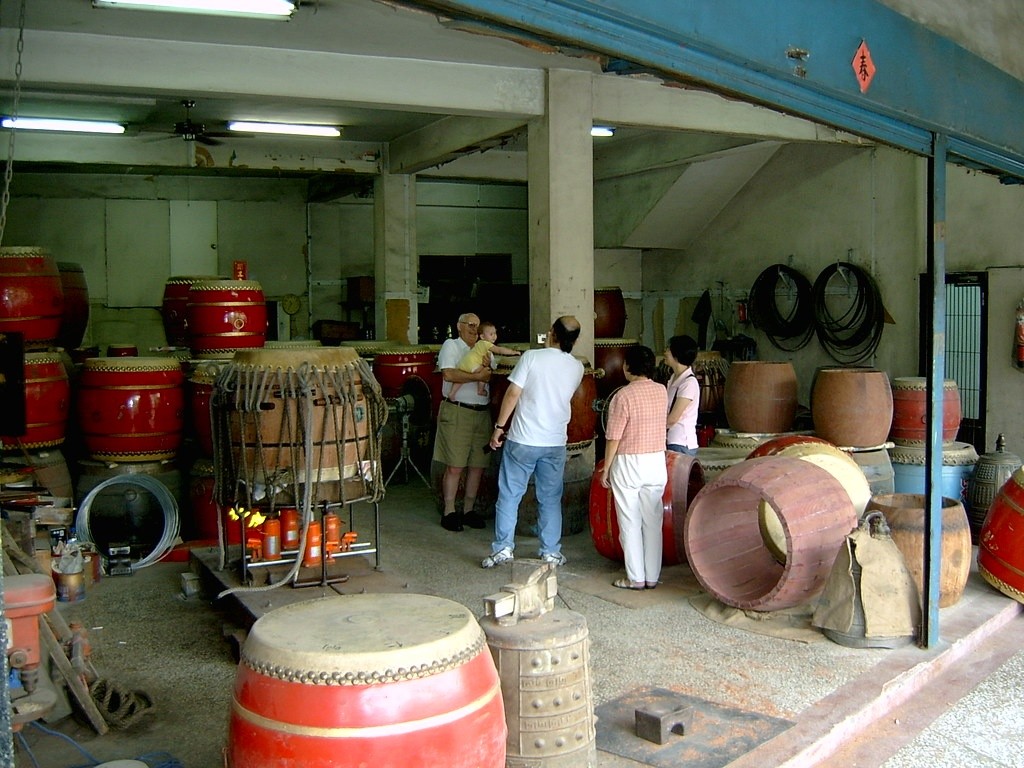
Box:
[226,120,345,137]
[592,126,615,137]
[0,114,128,134]
[90,0,304,21]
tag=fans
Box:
[126,99,256,146]
[383,375,433,490]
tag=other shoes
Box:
[463,510,487,530]
[441,510,464,532]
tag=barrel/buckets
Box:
[51,563,86,604]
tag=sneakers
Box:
[480,545,514,568]
[541,550,568,565]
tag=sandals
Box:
[646,578,657,590]
[613,576,647,589]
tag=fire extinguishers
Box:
[737,301,746,321]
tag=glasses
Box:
[462,322,477,328]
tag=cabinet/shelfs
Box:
[6,198,219,309]
[339,252,532,345]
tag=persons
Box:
[448,323,521,402]
[482,315,585,570]
[664,334,700,559]
[600,345,669,590]
[432,313,497,532]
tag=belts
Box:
[442,396,490,412]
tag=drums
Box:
[229,593,508,768]
[476,607,600,768]
[1,244,980,568]
[976,465,1024,604]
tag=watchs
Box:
[495,424,503,429]
